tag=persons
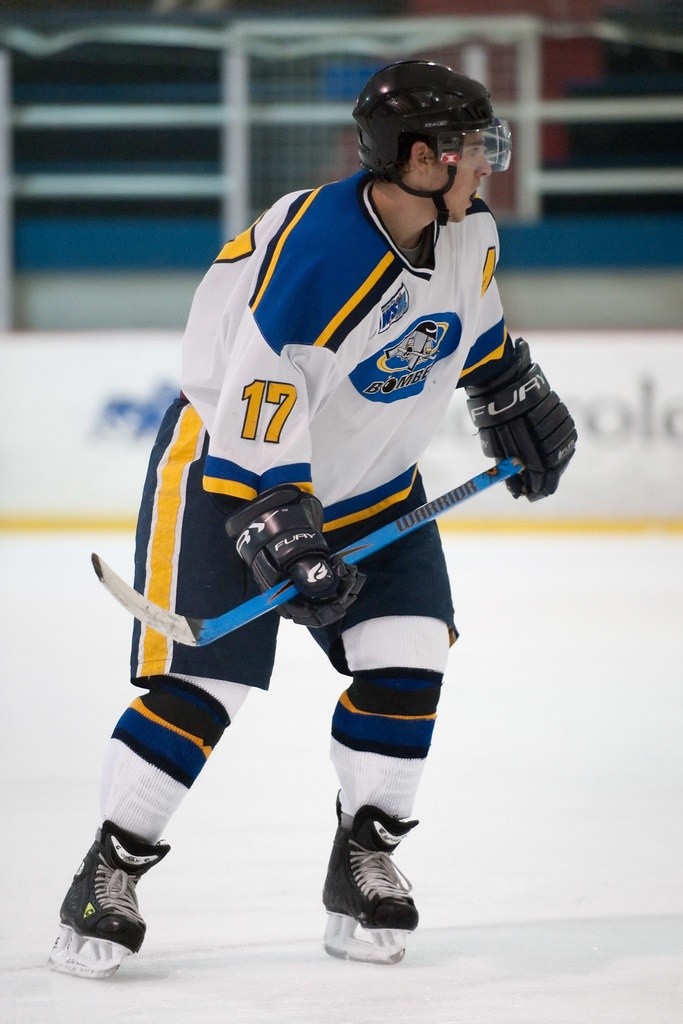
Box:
[48,60,578,978]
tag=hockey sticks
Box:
[91,448,531,650]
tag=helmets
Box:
[352,59,513,175]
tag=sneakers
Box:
[322,788,419,965]
[47,820,171,979]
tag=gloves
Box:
[225,484,366,628]
[465,337,577,502]
